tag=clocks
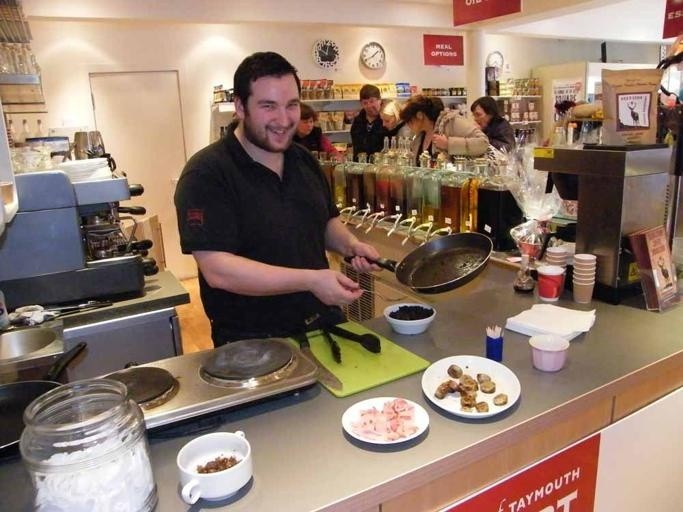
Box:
[485,49,504,74]
[360,40,386,69]
[312,37,340,70]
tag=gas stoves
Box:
[66,334,318,445]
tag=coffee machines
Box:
[1,169,157,304]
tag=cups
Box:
[511,221,598,308]
[485,336,503,360]
[528,333,571,373]
[383,300,436,336]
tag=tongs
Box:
[311,313,382,364]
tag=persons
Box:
[350,86,516,168]
[173,52,384,347]
[292,103,342,167]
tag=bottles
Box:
[554,120,595,148]
[1,40,41,76]
[7,118,51,149]
[320,133,495,235]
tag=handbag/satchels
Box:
[455,143,508,166]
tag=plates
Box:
[421,353,523,423]
[340,395,431,450]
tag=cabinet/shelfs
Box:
[211,94,542,163]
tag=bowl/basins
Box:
[173,432,255,507]
[1,328,66,383]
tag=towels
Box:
[9,303,61,327]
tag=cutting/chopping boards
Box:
[283,321,427,402]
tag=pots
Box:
[344,230,494,297]
[1,342,89,455]
